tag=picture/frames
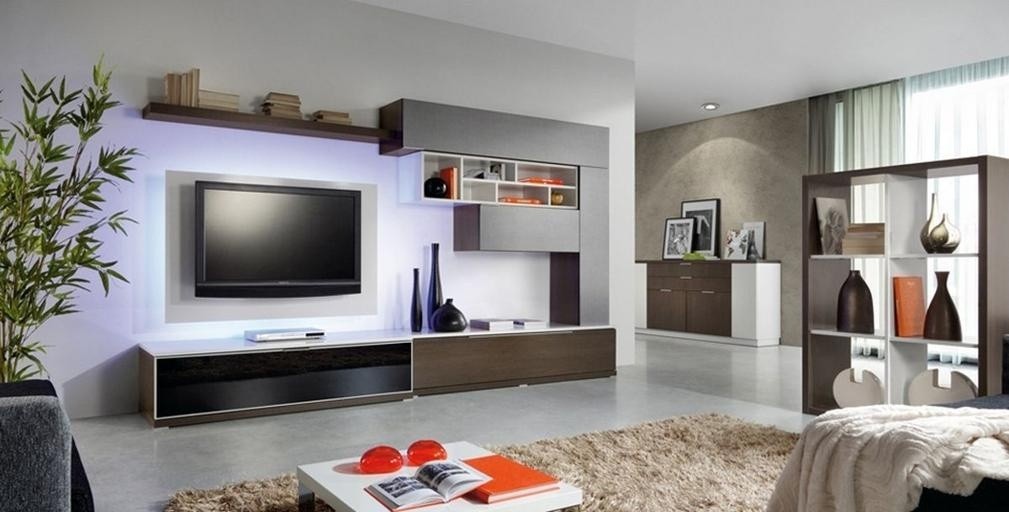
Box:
[662,199,720,260]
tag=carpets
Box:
[167,414,800,512]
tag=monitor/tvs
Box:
[194,180,362,300]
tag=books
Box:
[456,451,559,502]
[517,176,566,186]
[439,165,458,199]
[511,317,544,326]
[498,195,548,205]
[814,197,851,255]
[893,274,928,338]
[162,69,356,125]
[367,457,492,512]
[471,318,515,329]
[839,221,888,255]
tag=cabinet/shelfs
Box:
[802,155,1009,416]
[397,151,578,210]
[646,260,781,347]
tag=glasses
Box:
[356,438,446,474]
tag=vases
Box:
[918,193,961,253]
[411,242,467,332]
